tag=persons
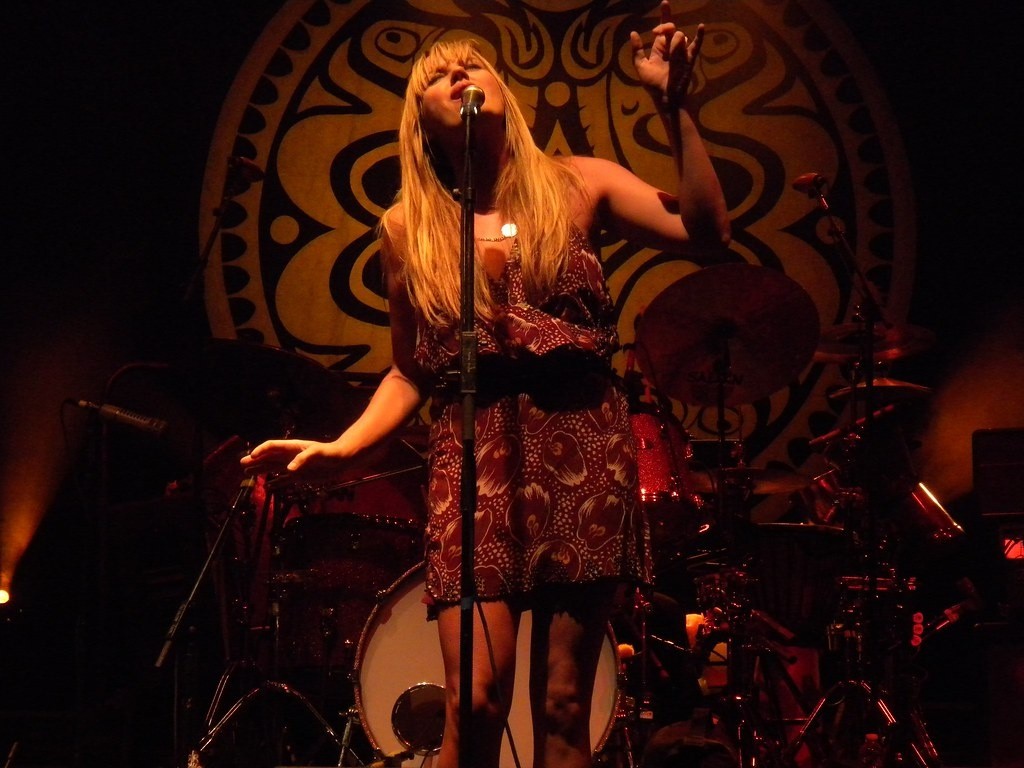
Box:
[239,19,731,768]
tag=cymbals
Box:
[633,263,821,408]
[177,340,353,441]
[830,376,929,402]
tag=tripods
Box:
[154,468,368,768]
[622,329,960,768]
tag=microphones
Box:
[66,398,168,438]
[809,404,894,446]
[791,172,827,193]
[227,156,265,181]
[461,85,485,120]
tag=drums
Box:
[903,481,964,550]
[185,429,429,677]
[351,557,622,768]
[741,521,848,652]
[273,517,427,667]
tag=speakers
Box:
[971,622,1024,768]
